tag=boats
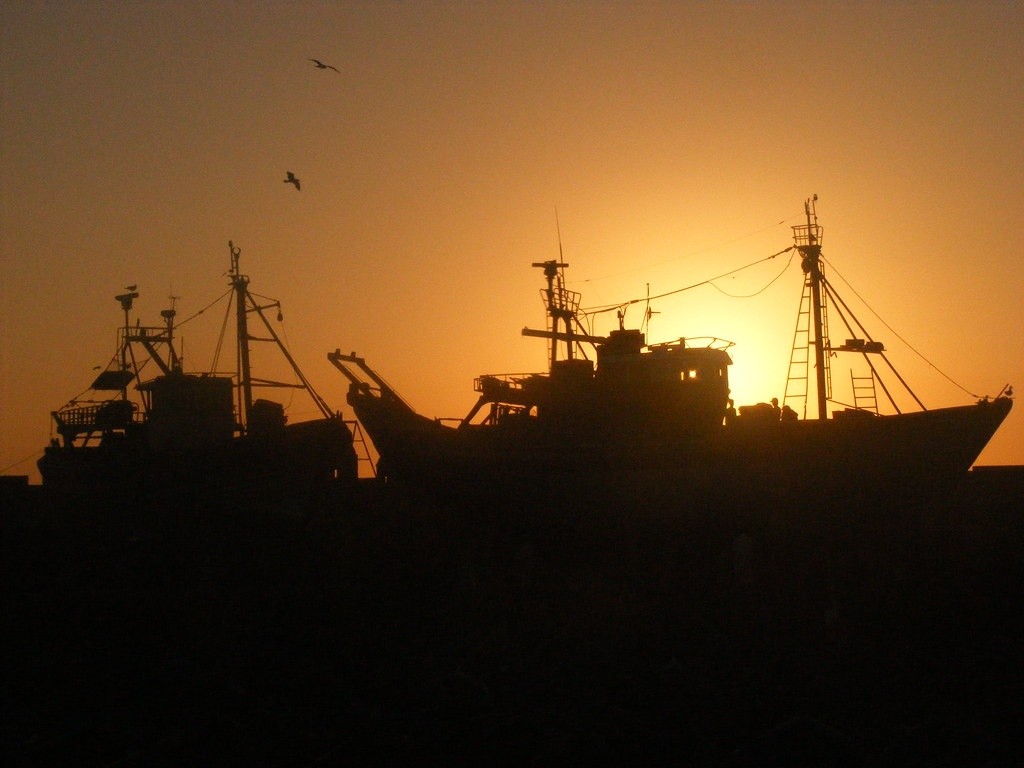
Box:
[324,196,1017,525]
[38,237,361,523]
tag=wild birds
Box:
[308,59,340,72]
[283,171,301,191]
